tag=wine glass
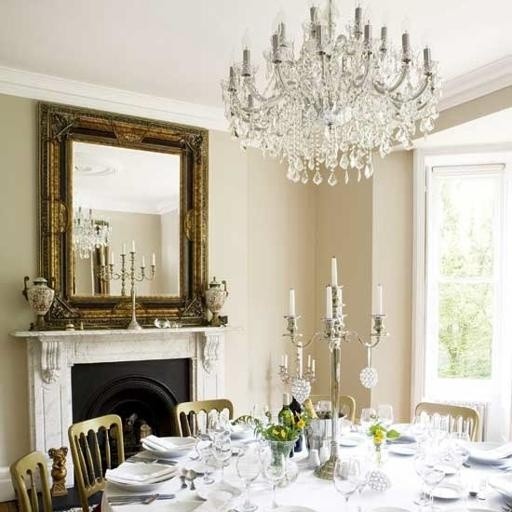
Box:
[184,392,484,512]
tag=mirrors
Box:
[36,101,208,327]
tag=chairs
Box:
[309,394,356,424]
[10,452,55,512]
[68,414,125,512]
[173,399,233,437]
[415,402,481,442]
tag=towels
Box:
[465,444,511,458]
[105,464,176,482]
[139,435,195,452]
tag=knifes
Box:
[108,493,175,506]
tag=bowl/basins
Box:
[104,461,182,492]
[486,471,512,507]
[140,434,197,459]
[461,440,512,470]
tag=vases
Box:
[265,441,299,487]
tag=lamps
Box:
[219,0,441,187]
[73,207,111,260]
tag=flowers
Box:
[254,407,306,466]
[367,423,400,445]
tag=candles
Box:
[100,253,105,267]
[121,242,125,255]
[110,254,114,265]
[141,255,145,268]
[131,240,135,251]
[274,254,389,375]
[151,253,155,265]
